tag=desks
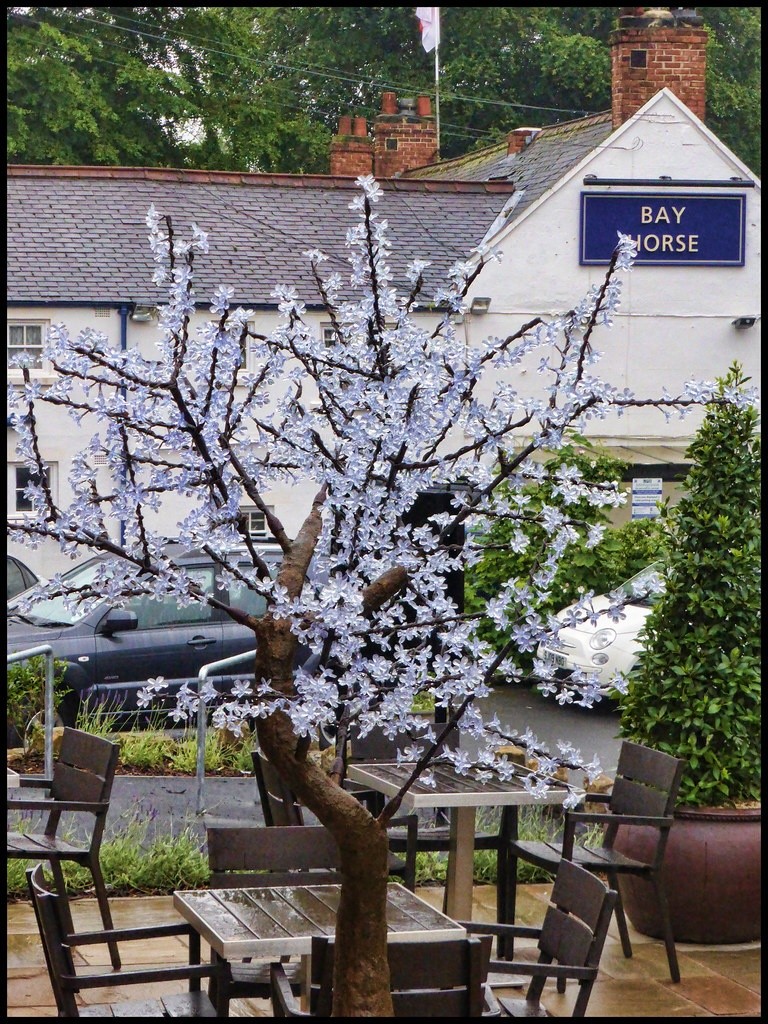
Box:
[349,760,584,922]
[170,882,467,1016]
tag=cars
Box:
[536,553,675,692]
[7,555,45,604]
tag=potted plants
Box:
[603,363,761,945]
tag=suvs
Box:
[7,536,335,735]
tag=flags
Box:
[415,7,440,53]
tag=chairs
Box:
[347,723,503,955]
[452,857,619,1017]
[207,825,344,1017]
[248,749,418,892]
[7,727,123,973]
[26,866,231,1016]
[502,742,686,982]
[270,933,492,1018]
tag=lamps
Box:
[129,301,158,322]
[470,297,491,315]
[732,318,756,329]
